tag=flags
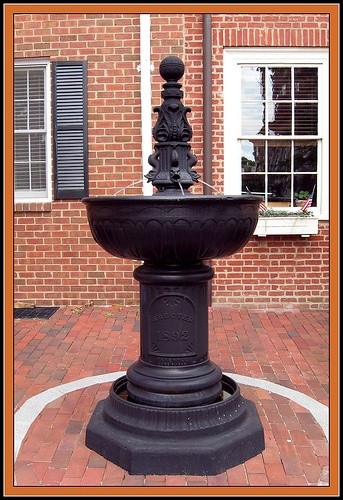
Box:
[248,190,267,213]
[300,189,314,212]
